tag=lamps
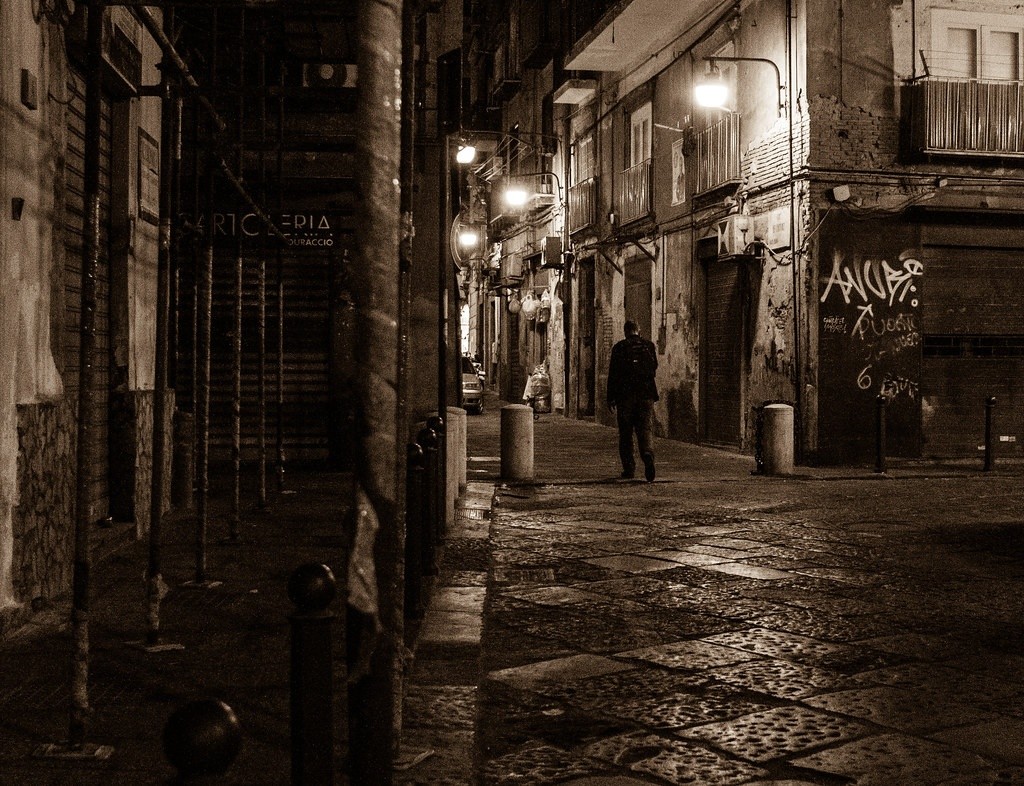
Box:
[696,56,784,118]
[502,172,563,203]
[456,129,559,164]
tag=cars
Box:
[462,357,486,414]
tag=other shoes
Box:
[643,456,656,481]
[621,471,634,478]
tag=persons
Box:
[605,319,660,482]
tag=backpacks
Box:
[623,338,656,385]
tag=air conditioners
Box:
[717,214,757,262]
[500,255,522,278]
[541,236,560,266]
